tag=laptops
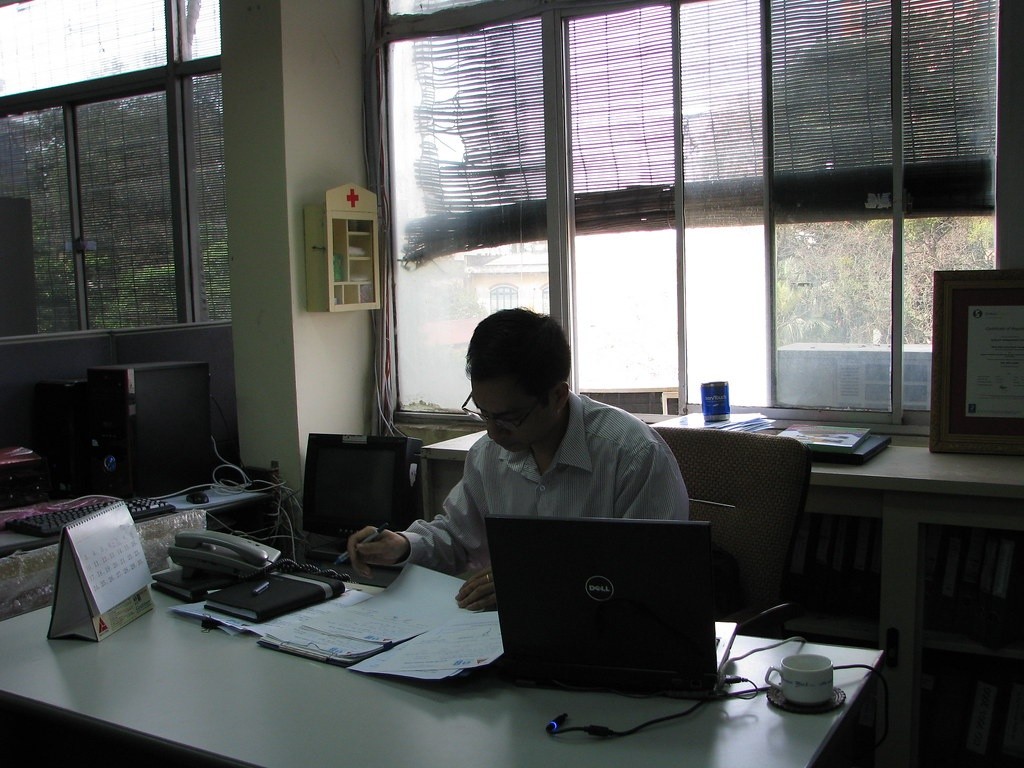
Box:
[483,513,738,701]
[812,435,892,465]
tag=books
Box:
[777,424,892,462]
[203,569,345,623]
[151,569,234,604]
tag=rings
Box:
[486,574,489,582]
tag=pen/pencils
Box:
[332,522,388,566]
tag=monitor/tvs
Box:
[300,432,424,554]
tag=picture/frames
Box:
[929,269,1024,455]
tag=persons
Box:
[346,308,688,611]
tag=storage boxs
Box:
[303,183,381,314]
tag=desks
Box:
[0,487,888,768]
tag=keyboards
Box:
[5,498,176,538]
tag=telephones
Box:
[167,530,280,579]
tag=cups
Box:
[765,654,833,702]
[701,382,730,422]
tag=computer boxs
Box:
[37,361,217,502]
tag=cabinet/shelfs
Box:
[421,413,1024,768]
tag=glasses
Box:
[462,376,566,431]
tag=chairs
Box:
[651,428,812,639]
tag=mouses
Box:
[187,492,210,505]
